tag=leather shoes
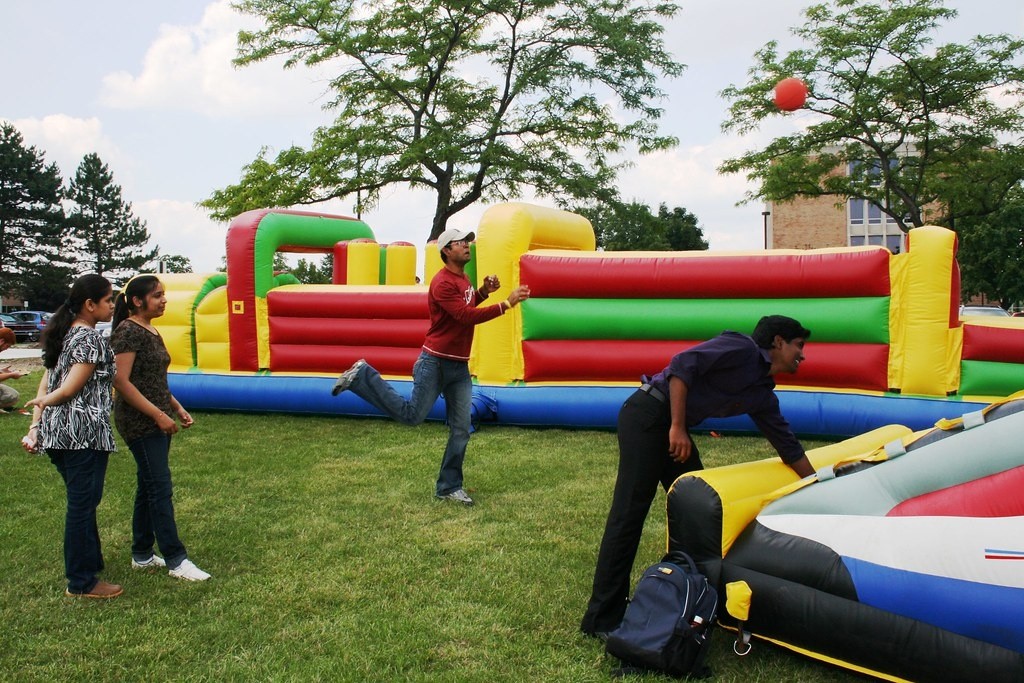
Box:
[65,581,123,598]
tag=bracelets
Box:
[503,300,511,308]
[30,424,37,429]
[155,411,166,424]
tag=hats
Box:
[437,230,475,253]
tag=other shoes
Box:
[584,630,618,644]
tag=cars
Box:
[0,313,38,345]
[9,310,55,342]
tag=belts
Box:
[639,383,666,403]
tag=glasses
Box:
[448,240,470,247]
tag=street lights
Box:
[761,212,771,250]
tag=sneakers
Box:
[131,554,166,567]
[332,359,366,396]
[436,489,473,505]
[170,560,212,582]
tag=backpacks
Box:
[605,551,720,680]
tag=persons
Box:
[0,328,22,414]
[416,276,420,283]
[22,274,212,598]
[580,315,818,634]
[331,229,530,506]
